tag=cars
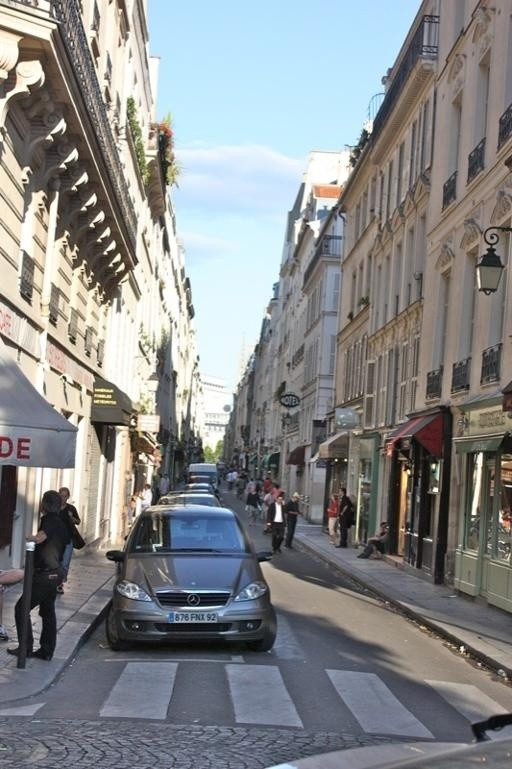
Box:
[105,502,282,652]
[155,474,224,519]
[467,512,512,562]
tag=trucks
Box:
[186,463,220,491]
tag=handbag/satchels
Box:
[74,526,85,549]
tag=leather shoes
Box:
[32,648,51,660]
[7,647,33,658]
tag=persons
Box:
[55,486,82,594]
[498,504,511,532]
[221,465,304,556]
[156,474,168,498]
[334,488,352,548]
[141,483,153,509]
[183,467,187,482]
[5,490,73,661]
[355,520,392,558]
[325,493,339,545]
[1,568,27,641]
[128,493,137,524]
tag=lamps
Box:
[473,226,511,295]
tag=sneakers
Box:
[0,625,8,641]
[56,584,64,594]
